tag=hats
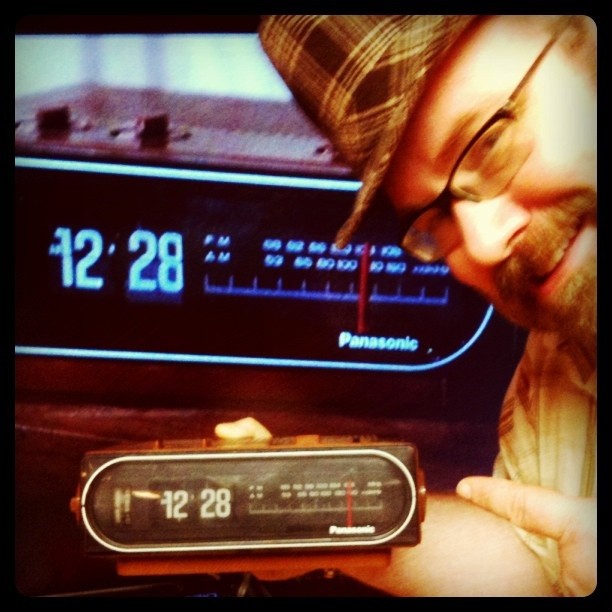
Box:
[257,15,475,251]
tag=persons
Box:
[213,15,597,597]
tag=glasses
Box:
[398,14,576,265]
